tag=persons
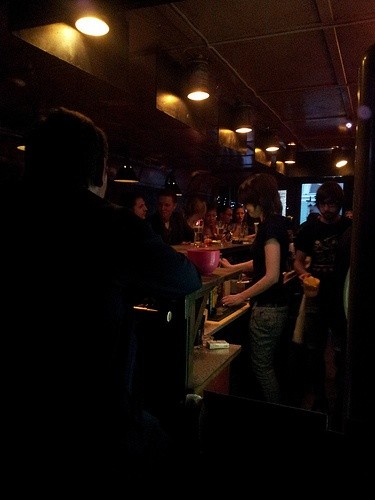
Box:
[221,172,302,404]
[294,182,352,411]
[115,189,256,246]
[0,105,199,500]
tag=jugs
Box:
[302,276,320,296]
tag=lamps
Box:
[333,146,348,168]
[181,45,214,100]
[266,113,282,152]
[236,93,256,134]
[284,133,296,164]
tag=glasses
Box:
[316,200,337,208]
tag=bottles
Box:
[193,217,203,248]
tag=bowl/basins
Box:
[188,250,220,274]
